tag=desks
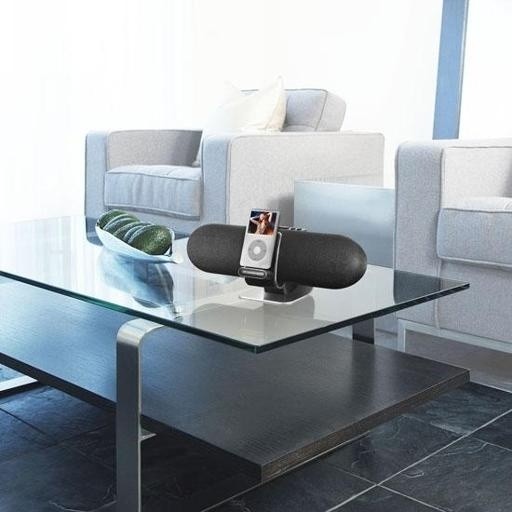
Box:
[293,171,398,335]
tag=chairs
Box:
[83,91,384,245]
[394,139,512,392]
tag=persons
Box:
[250,211,272,235]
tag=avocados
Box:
[96,208,175,256]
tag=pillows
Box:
[192,82,287,167]
